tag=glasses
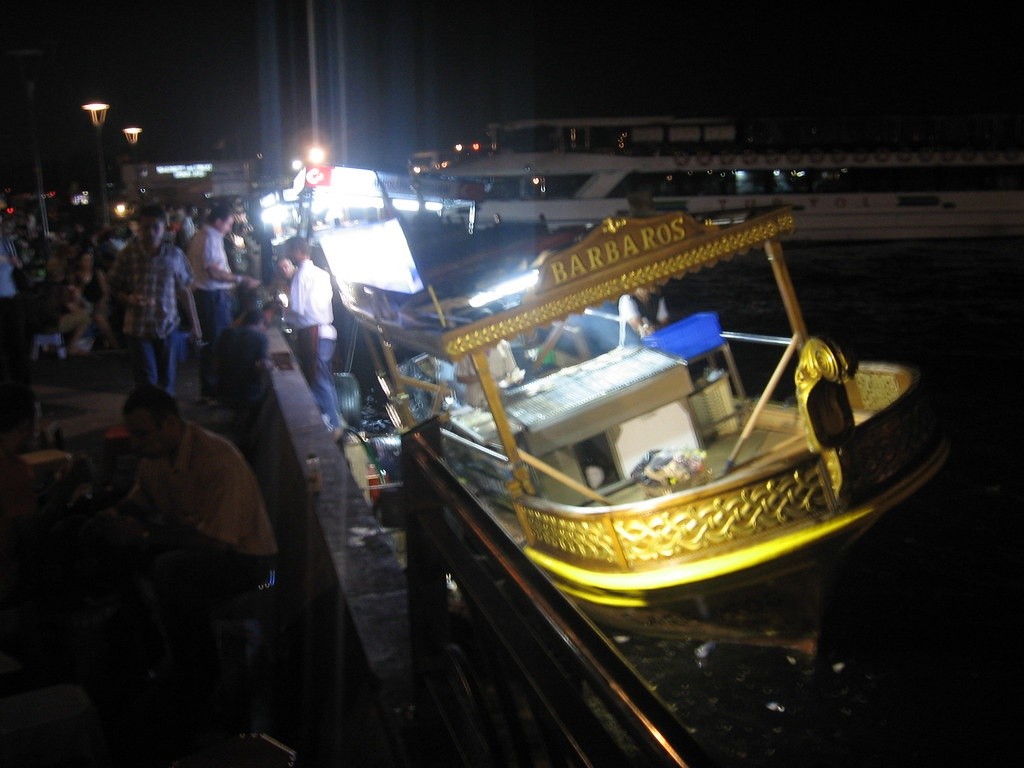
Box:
[133,427,157,438]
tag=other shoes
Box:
[26,406,57,422]
[68,348,92,360]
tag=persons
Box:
[0,191,338,697]
[454,324,525,406]
[618,286,668,347]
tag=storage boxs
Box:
[643,313,725,361]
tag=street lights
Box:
[82,100,110,231]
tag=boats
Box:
[319,162,951,654]
[386,114,1024,247]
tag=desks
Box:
[682,342,754,449]
